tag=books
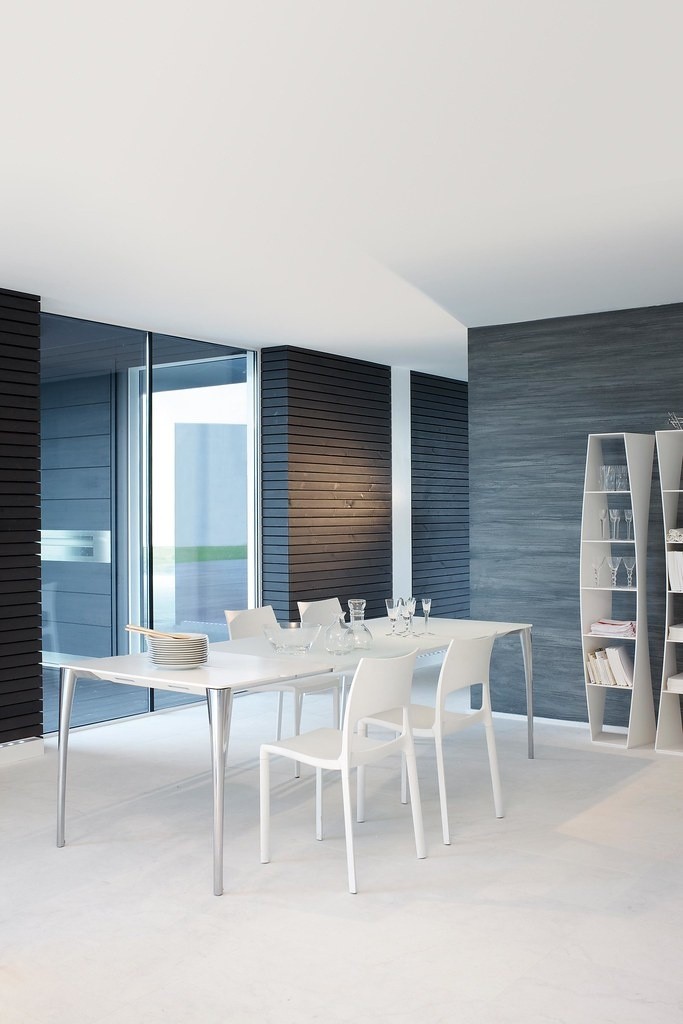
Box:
[667,622,683,641]
[586,644,633,688]
[666,671,683,693]
[589,618,636,638]
[662,551,683,593]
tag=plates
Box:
[145,633,209,670]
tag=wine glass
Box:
[385,596,435,638]
[590,556,637,589]
[597,508,633,541]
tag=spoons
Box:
[125,624,191,639]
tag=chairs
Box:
[296,596,369,739]
[357,630,505,846]
[224,604,340,778]
[260,647,428,894]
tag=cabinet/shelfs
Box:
[579,432,657,749]
[654,429,683,755]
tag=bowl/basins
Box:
[262,622,322,655]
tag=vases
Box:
[343,600,374,649]
[324,611,356,655]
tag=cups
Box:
[599,465,629,491]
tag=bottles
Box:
[343,599,374,651]
[324,611,355,656]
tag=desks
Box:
[38,616,536,896]
